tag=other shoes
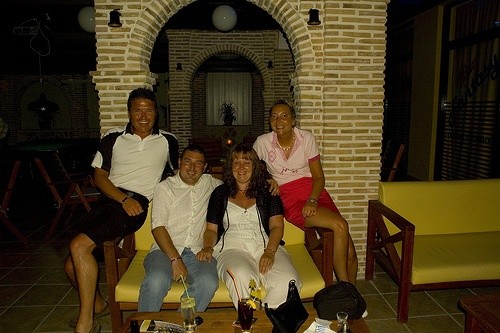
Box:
[74,319,102,333]
[69,300,112,328]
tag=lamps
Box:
[27,50,59,111]
[78,3,95,32]
[267,60,273,68]
[176,63,182,70]
[307,8,321,25]
[212,5,237,32]
[108,9,122,27]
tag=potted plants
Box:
[217,101,236,126]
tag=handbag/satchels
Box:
[264,279,309,333]
[313,280,367,320]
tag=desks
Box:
[457,293,500,333]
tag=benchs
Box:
[365,179,500,324]
[104,201,334,333]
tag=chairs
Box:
[29,149,100,239]
[0,159,26,246]
[188,136,222,173]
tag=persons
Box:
[137,144,280,313]
[252,101,359,284]
[196,143,304,312]
[65,87,183,333]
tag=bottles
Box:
[130,320,140,333]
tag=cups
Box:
[237,298,253,333]
[181,295,197,332]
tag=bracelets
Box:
[307,198,318,204]
[171,257,182,262]
[121,195,130,206]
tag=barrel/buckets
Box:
[127,321,185,333]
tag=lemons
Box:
[246,300,256,309]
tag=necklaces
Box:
[238,189,247,192]
[276,128,296,150]
